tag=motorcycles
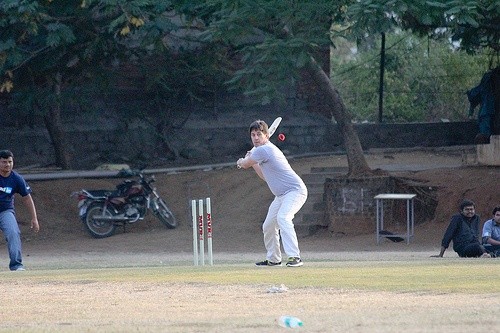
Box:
[77,159,179,239]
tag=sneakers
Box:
[286,257,304,266]
[255,259,282,267]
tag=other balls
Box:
[277,134,285,140]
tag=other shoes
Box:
[15,268,25,271]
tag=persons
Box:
[482,206,500,259]
[236,120,309,267]
[0,149,39,271]
[429,199,497,258]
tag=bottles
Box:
[277,316,302,328]
